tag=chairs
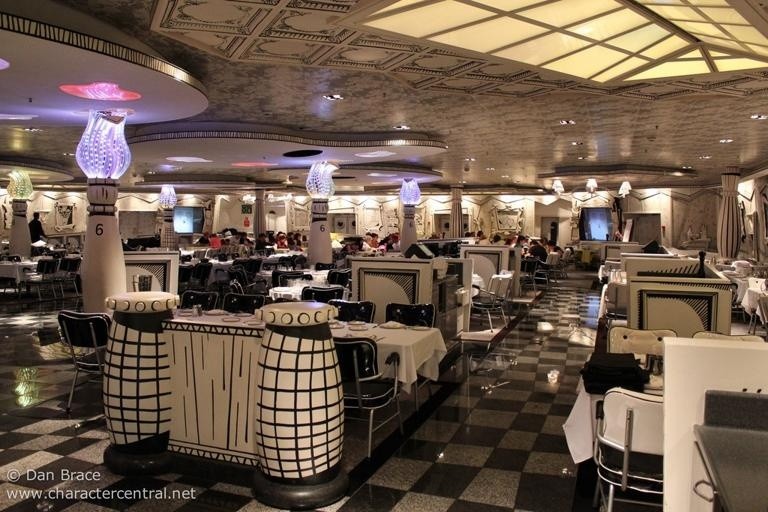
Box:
[1,229,768,510]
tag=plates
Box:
[179,307,429,345]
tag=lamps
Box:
[399,176,421,206]
[306,160,341,200]
[77,112,134,179]
[159,183,177,209]
[6,167,34,199]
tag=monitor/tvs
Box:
[405,242,436,259]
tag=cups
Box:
[192,304,205,317]
[609,268,625,284]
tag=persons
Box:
[28,212,48,257]
[336,232,400,269]
[198,228,308,252]
[464,230,563,281]
